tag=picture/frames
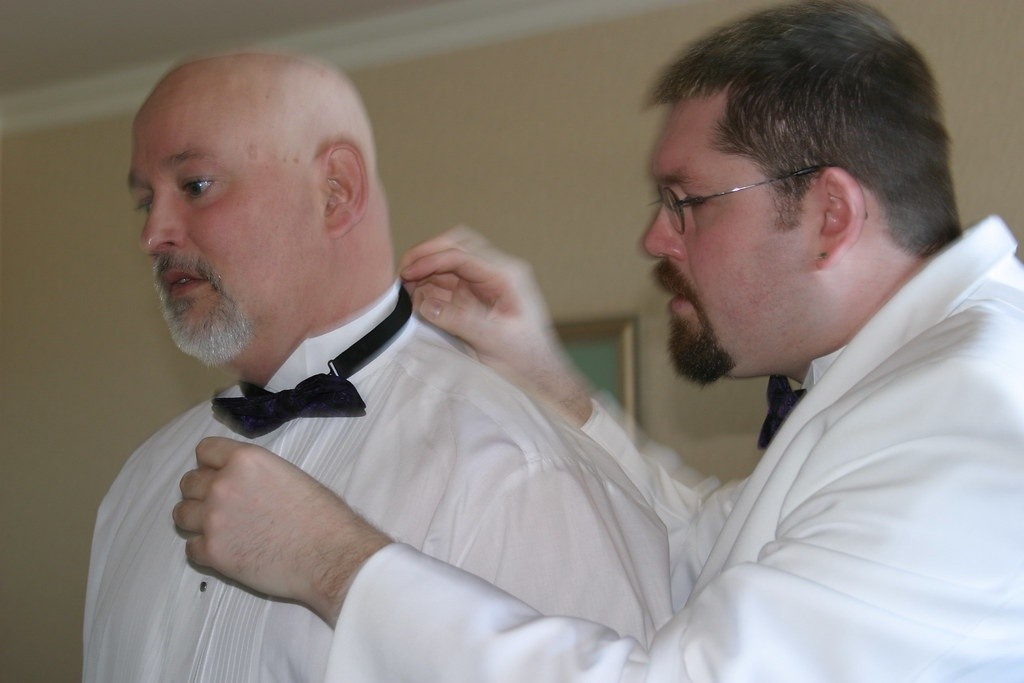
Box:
[550,313,642,438]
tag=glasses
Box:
[657,165,868,234]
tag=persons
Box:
[170,0,1024,682]
[75,52,674,682]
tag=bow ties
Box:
[757,375,808,448]
[212,286,412,439]
